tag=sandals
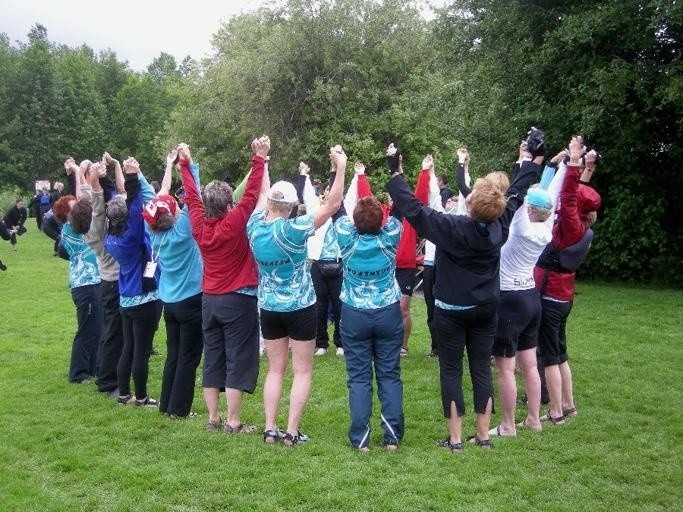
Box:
[437,391,577,455]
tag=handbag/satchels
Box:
[318,262,342,276]
[142,261,161,290]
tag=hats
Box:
[528,186,554,209]
[269,180,299,203]
[578,183,600,214]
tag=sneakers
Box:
[206,417,310,448]
[68,372,201,419]
[356,439,400,450]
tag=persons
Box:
[1,198,27,251]
[28,127,601,452]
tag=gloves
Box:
[529,129,547,158]
[387,150,400,174]
